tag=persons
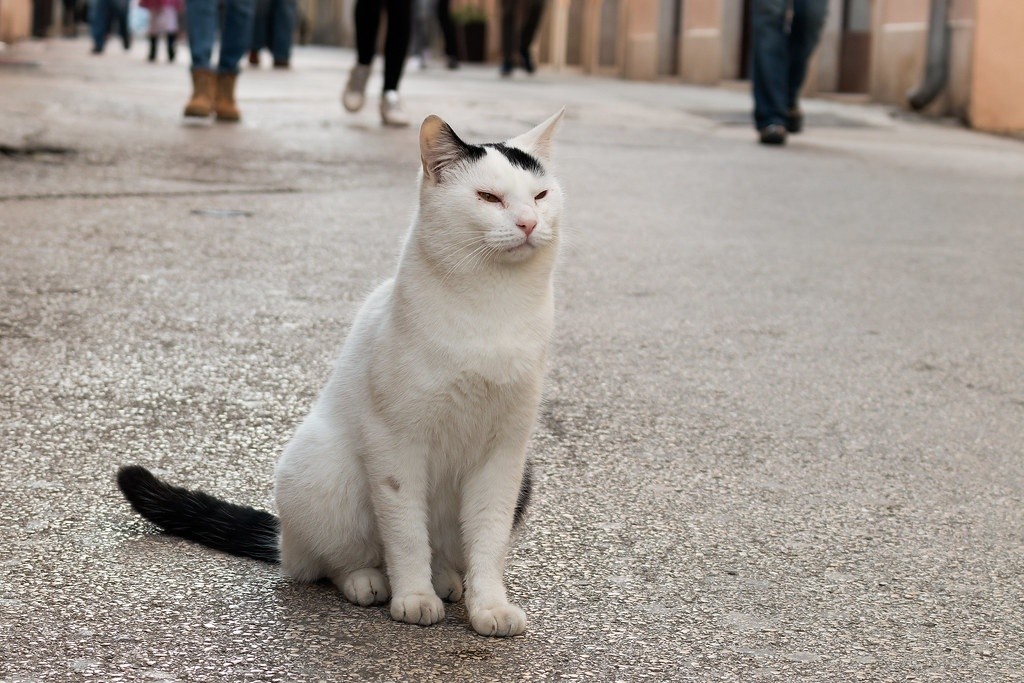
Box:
[497,0,548,76]
[86,0,132,55]
[247,0,297,72]
[184,0,254,122]
[407,1,463,72]
[137,0,179,61]
[749,1,828,146]
[342,0,417,127]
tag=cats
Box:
[114,106,571,633]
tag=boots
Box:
[214,76,239,120]
[185,68,216,116]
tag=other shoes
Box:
[343,66,369,111]
[382,90,409,124]
[787,108,802,132]
[761,124,786,143]
